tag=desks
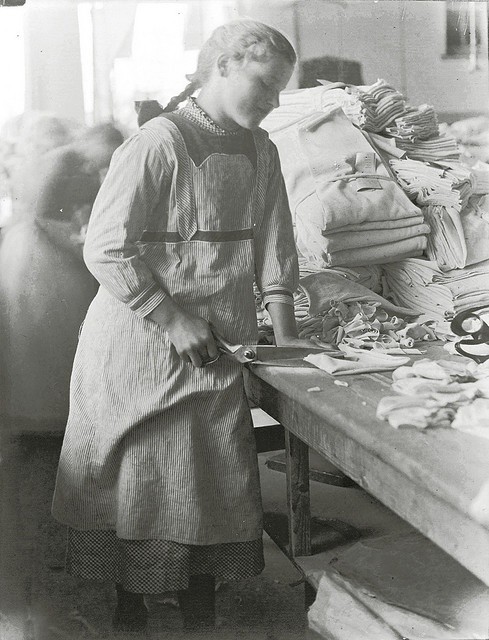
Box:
[242,340,489,585]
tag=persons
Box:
[52,19,344,633]
[3,124,125,455]
